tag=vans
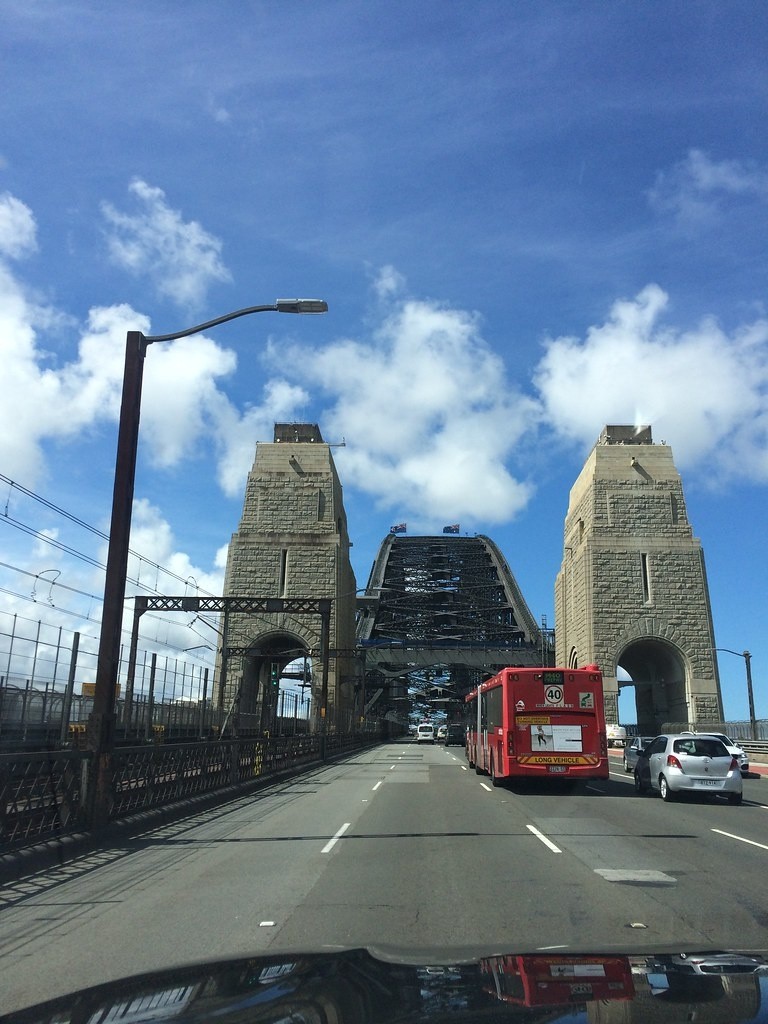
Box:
[417,724,435,745]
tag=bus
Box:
[462,663,610,786]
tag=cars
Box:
[632,734,743,803]
[680,730,749,778]
[623,736,656,773]
[437,726,448,741]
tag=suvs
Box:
[444,725,467,748]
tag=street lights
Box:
[706,647,759,741]
[83,297,328,825]
